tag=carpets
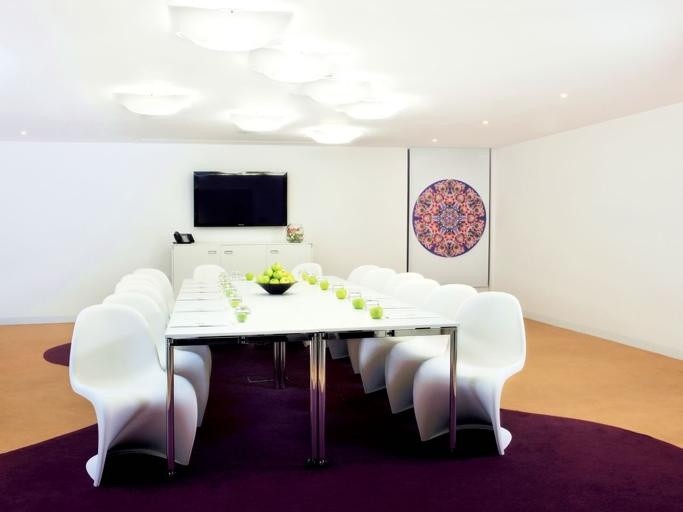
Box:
[0,335,683,510]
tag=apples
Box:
[309,275,316,284]
[245,272,254,280]
[370,303,384,319]
[303,272,308,280]
[320,280,329,290]
[257,263,294,284]
[237,312,247,322]
[335,288,347,299]
[231,299,240,307]
[352,296,365,309]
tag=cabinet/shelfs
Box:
[171,242,315,290]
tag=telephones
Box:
[173,232,194,244]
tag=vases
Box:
[286,223,303,243]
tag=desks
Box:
[166,263,460,478]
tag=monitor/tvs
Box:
[194,171,287,227]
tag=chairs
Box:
[328,267,479,414]
[103,268,212,428]
[68,304,199,487]
[414,291,526,456]
